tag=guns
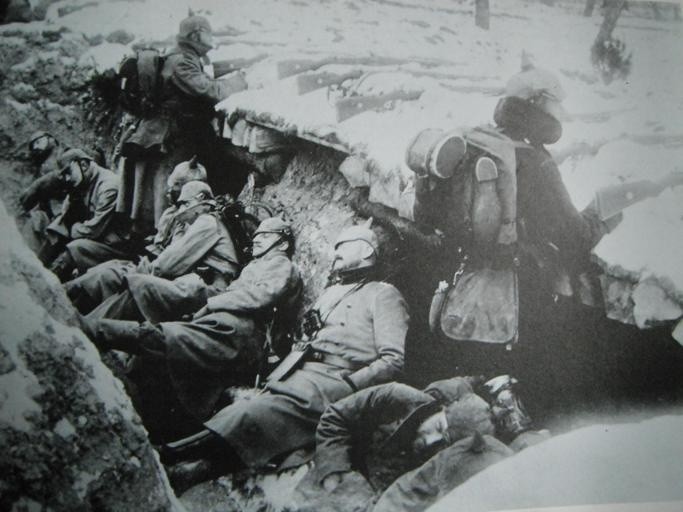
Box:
[211,51,272,79]
[595,173,681,221]
[277,57,509,124]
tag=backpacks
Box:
[117,46,202,120]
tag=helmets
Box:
[180,7,212,39]
[251,211,292,239]
[334,216,380,259]
[57,148,94,176]
[27,130,59,151]
[166,154,208,192]
[445,391,511,446]
[493,48,567,144]
[176,180,215,204]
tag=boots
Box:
[153,429,222,466]
[161,451,219,498]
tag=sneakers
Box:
[460,155,503,258]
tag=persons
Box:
[18,16,624,512]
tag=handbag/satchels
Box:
[440,265,520,351]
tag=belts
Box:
[302,350,368,371]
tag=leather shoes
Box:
[404,122,535,247]
